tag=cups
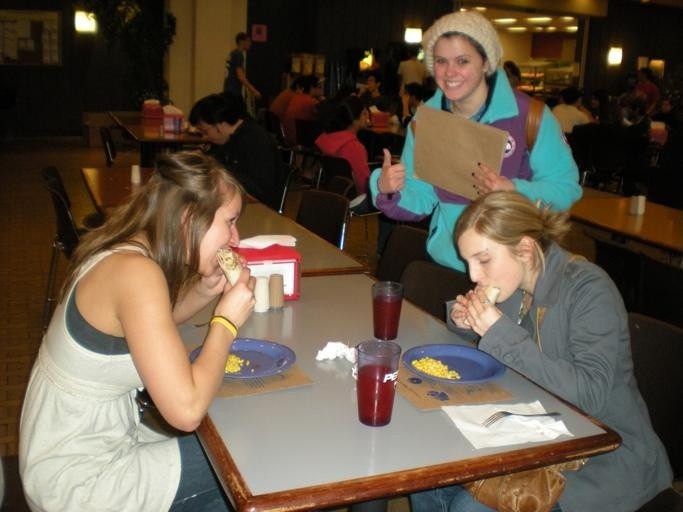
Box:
[369,279,403,340]
[356,338,399,428]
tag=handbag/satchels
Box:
[463,456,593,512]
[132,385,196,440]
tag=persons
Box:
[186,92,290,217]
[17,145,257,511]
[407,189,676,512]
[368,10,585,274]
[221,31,683,210]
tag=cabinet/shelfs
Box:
[518,60,552,93]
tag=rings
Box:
[480,298,491,305]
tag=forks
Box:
[480,410,564,424]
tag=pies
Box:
[216,245,244,287]
[463,284,499,328]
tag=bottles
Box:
[173,116,182,135]
[629,193,647,216]
[130,165,141,185]
[159,121,164,137]
[251,271,286,315]
[252,310,281,341]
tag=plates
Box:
[403,344,506,386]
[192,337,298,381]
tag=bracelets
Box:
[208,314,239,340]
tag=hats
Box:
[418,7,504,80]
